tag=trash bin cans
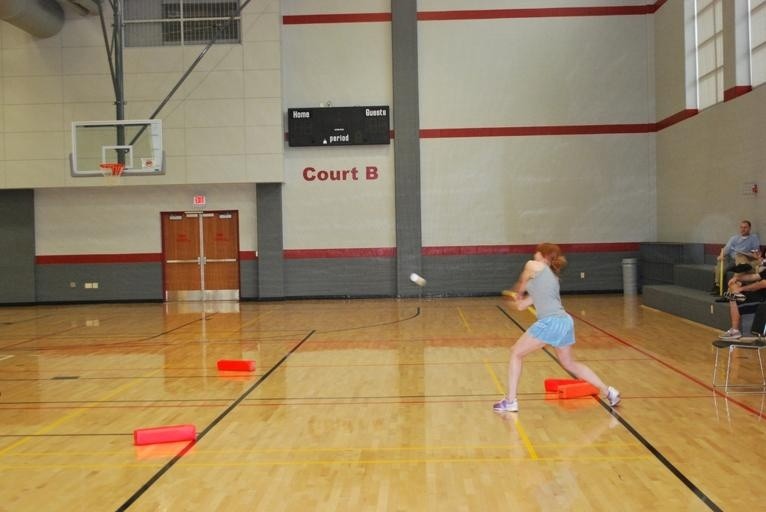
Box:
[622,258,638,292]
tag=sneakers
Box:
[606,386,622,407]
[712,286,726,291]
[493,398,519,412]
[723,290,747,304]
[734,264,752,273]
[710,291,720,295]
[718,327,742,338]
[715,297,730,302]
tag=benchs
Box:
[640,262,754,338]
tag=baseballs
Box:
[409,273,427,287]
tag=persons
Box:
[710,220,766,338]
[492,242,621,412]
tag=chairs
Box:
[712,303,766,394]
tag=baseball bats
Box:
[720,248,723,297]
[501,288,536,316]
[731,245,762,260]
